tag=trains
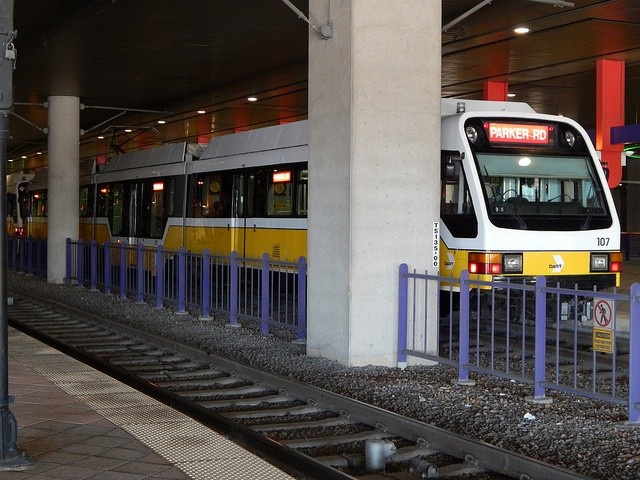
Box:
[2,99,622,326]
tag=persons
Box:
[212,200,221,215]
